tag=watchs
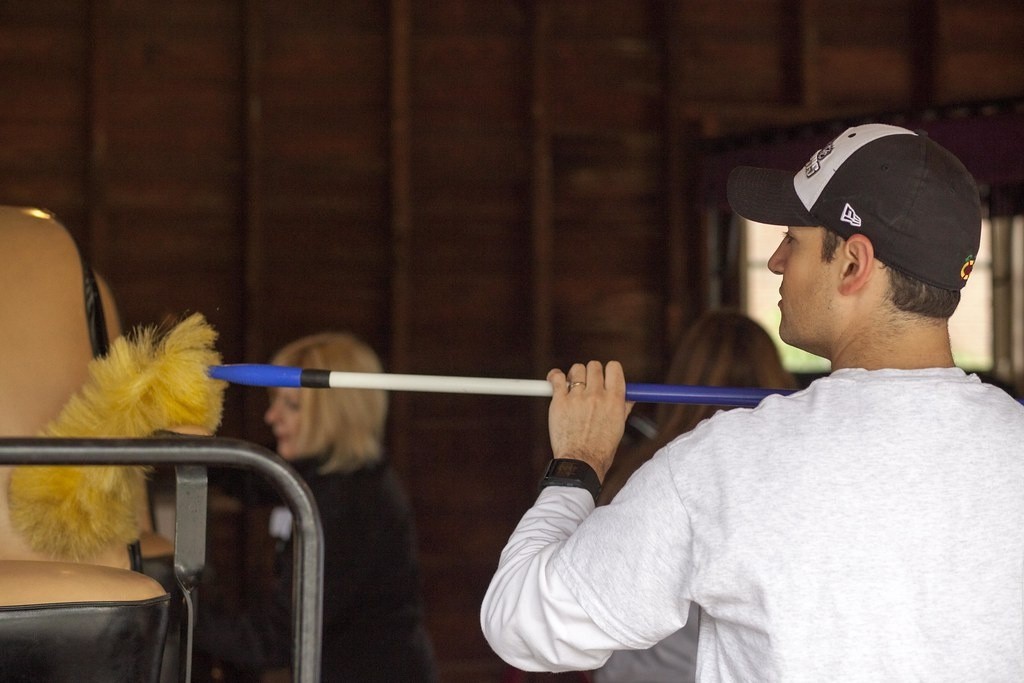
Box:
[539,458,602,503]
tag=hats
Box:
[725,119,982,287]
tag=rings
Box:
[570,382,586,390]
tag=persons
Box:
[480,124,1024,683]
[222,332,430,683]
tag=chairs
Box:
[0,200,214,683]
